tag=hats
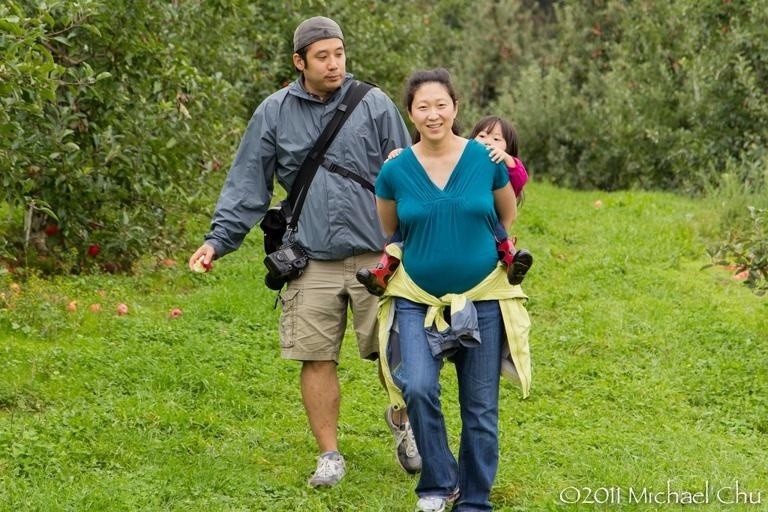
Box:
[292,15,344,72]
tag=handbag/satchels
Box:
[259,199,288,255]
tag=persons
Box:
[353,116,533,296]
[188,16,423,492]
[369,69,516,511]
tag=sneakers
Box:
[306,448,347,490]
[414,485,461,512]
[384,404,422,475]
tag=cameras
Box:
[263,242,309,291]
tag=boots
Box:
[496,238,534,286]
[355,241,400,297]
